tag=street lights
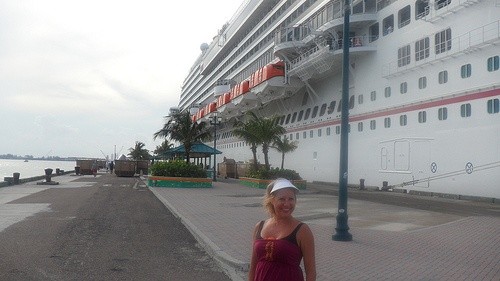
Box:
[209,112,223,181]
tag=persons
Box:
[350,22,394,47]
[247,177,319,281]
[90,159,116,178]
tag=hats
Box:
[270,179,301,194]
